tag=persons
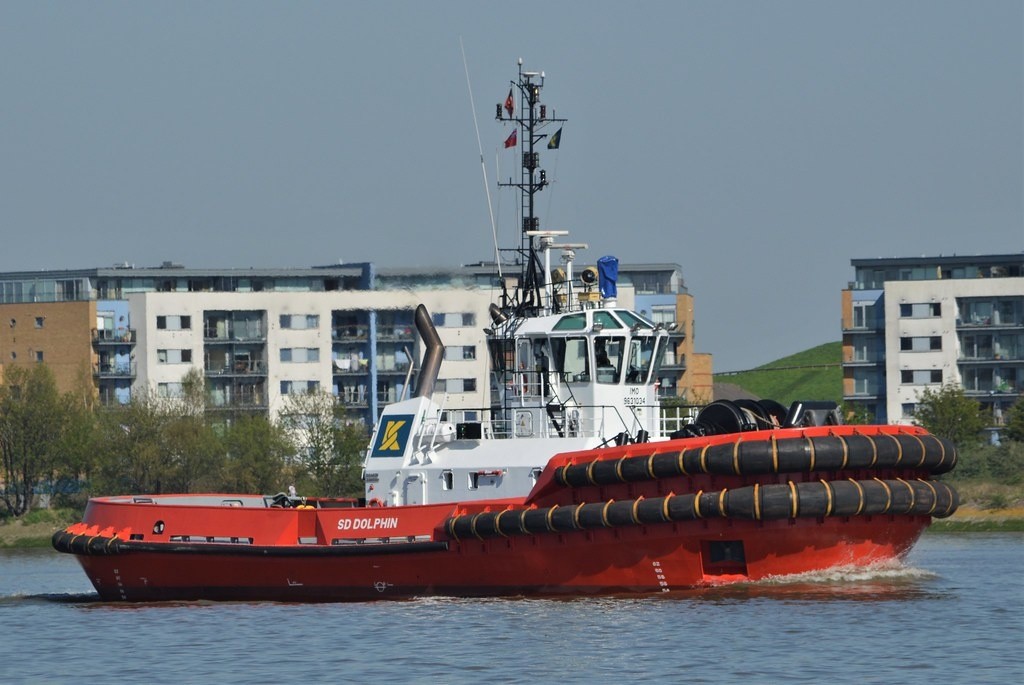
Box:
[599,351,611,367]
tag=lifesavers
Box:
[368,498,384,507]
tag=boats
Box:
[50,59,960,603]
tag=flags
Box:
[505,129,517,148]
[505,89,514,119]
[548,127,562,149]
